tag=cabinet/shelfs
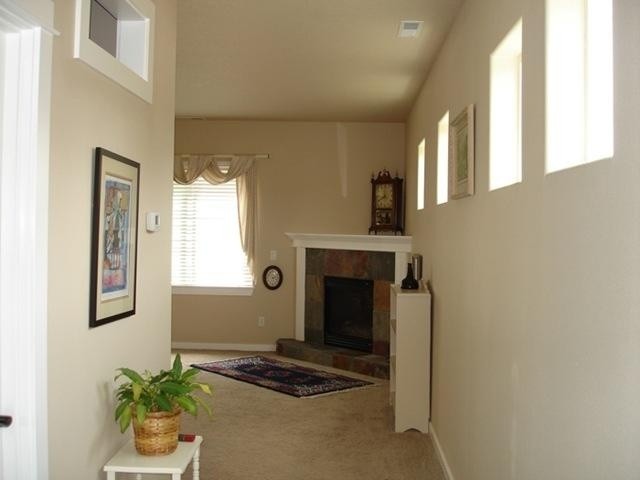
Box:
[389,282,431,434]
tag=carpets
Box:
[189,355,377,400]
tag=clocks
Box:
[368,167,405,235]
[263,265,283,290]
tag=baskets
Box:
[132,403,181,456]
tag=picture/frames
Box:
[88,147,141,328]
[448,103,473,200]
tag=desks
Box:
[103,436,205,480]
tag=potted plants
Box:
[112,352,215,458]
